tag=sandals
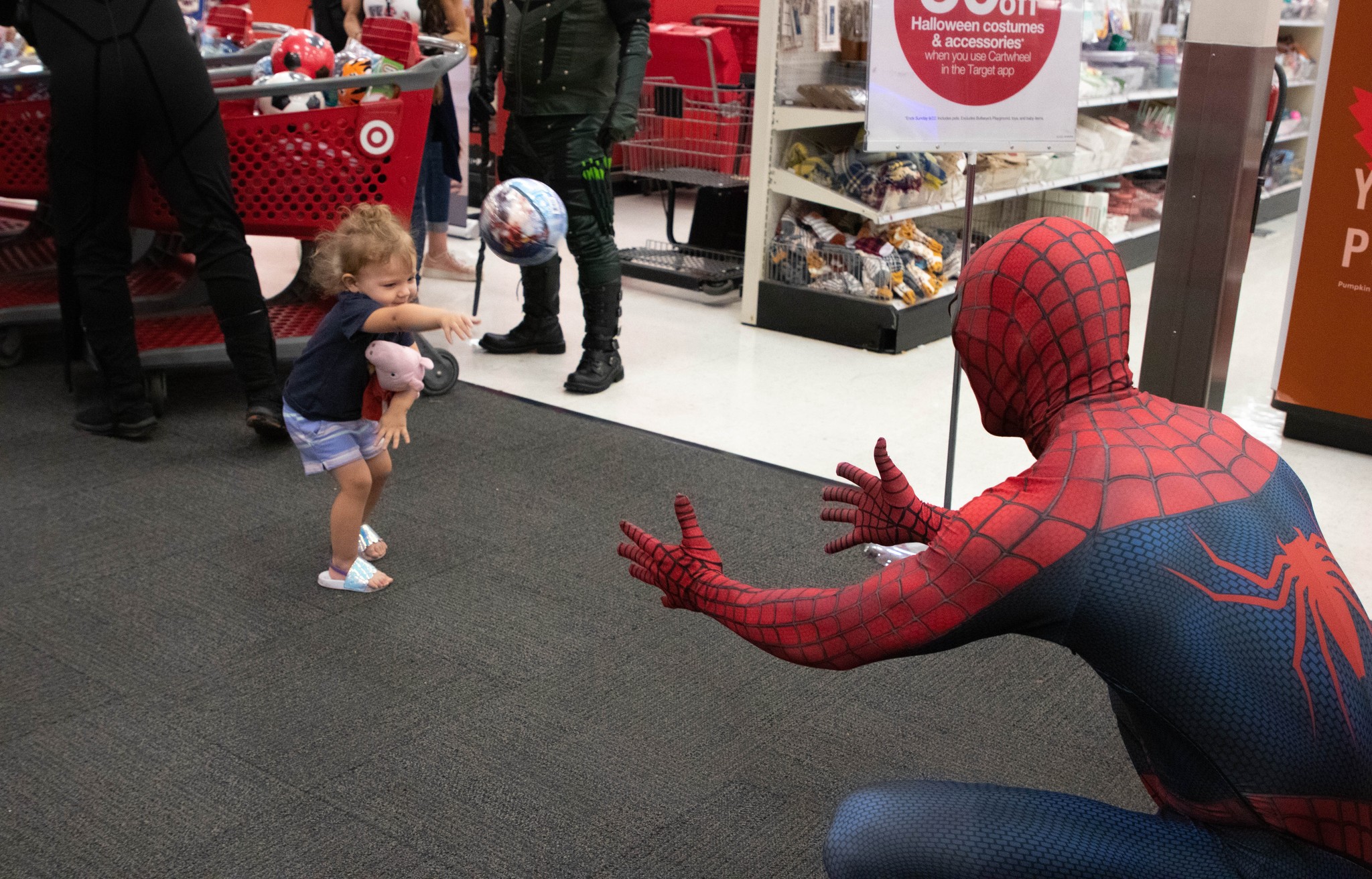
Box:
[357,523,387,561]
[317,556,394,593]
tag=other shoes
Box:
[564,347,627,393]
[479,316,567,354]
[246,403,291,441]
[74,404,155,440]
[422,252,485,281]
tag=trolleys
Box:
[0,5,467,355]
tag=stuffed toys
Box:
[360,339,435,419]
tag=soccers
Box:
[255,70,323,118]
[270,29,334,83]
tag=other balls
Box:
[475,177,569,267]
[336,58,374,104]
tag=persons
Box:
[281,203,483,593]
[616,214,1372,879]
[0,1,294,447]
[307,0,481,282]
[469,0,652,393]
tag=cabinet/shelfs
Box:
[739,0,1331,355]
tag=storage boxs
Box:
[360,59,404,104]
[780,110,1133,212]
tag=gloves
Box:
[470,87,496,123]
